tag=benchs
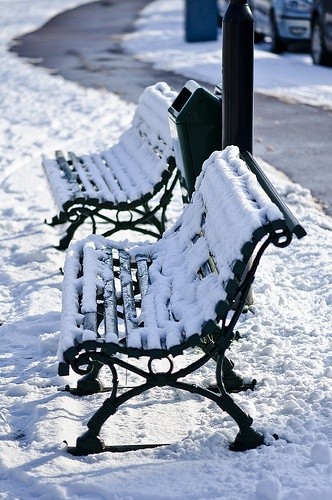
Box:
[57,147,307,458]
[40,82,179,253]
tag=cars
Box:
[217,0,332,67]
